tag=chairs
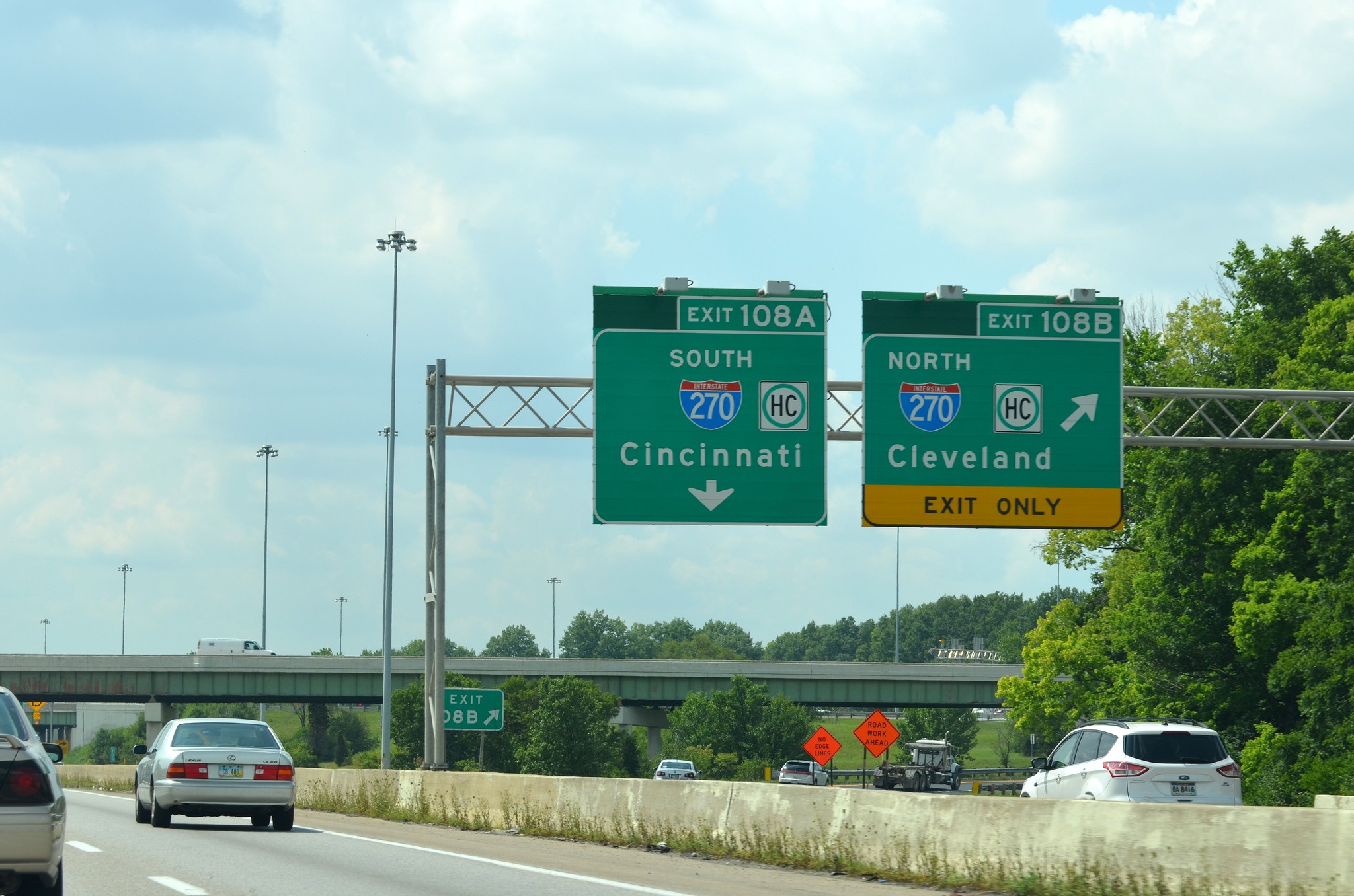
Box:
[237,736,262,746]
[180,737,204,746]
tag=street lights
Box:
[40,619,50,654]
[547,578,561,659]
[378,228,419,770]
[335,596,347,656]
[255,445,279,731]
[117,564,133,654]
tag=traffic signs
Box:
[863,335,1125,532]
[443,687,504,731]
[56,740,68,754]
[591,329,829,527]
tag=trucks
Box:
[197,639,276,655]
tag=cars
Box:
[994,710,1012,714]
[1,686,66,896]
[133,717,296,830]
[779,760,830,787]
[652,759,703,781]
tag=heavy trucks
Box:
[873,732,962,792]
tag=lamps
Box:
[657,277,694,296]
[925,286,968,301]
[1057,289,1101,305]
[759,280,796,296]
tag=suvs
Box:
[1020,719,1242,806]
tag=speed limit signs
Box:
[33,712,41,720]
